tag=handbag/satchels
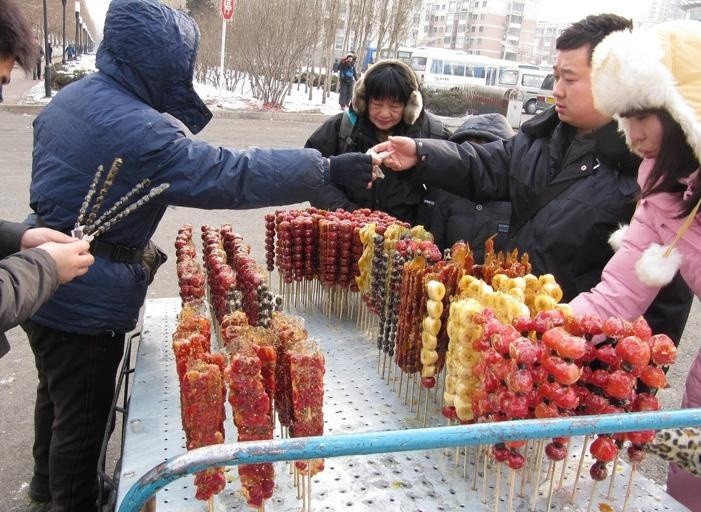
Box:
[141,240,167,285]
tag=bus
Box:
[360,39,540,97]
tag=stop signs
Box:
[220,0,236,20]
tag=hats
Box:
[590,19,701,163]
[347,53,354,59]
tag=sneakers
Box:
[29,475,111,503]
[341,104,350,111]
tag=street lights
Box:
[42,1,96,98]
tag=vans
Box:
[498,68,546,114]
[535,71,556,110]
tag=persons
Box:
[556,18,701,511]
[366,12,691,349]
[65,44,76,59]
[333,60,340,73]
[1,1,96,358]
[428,111,512,274]
[301,57,453,235]
[32,36,45,82]
[48,43,53,64]
[339,51,360,110]
[15,1,386,512]
